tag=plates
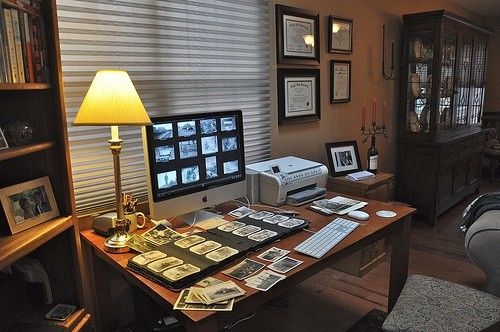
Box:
[443,76,453,91]
[426,111,430,127]
[409,36,423,59]
[427,74,432,91]
[410,112,418,132]
[440,108,450,124]
[409,73,420,97]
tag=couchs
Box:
[464,207,500,292]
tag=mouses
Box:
[348,211,369,220]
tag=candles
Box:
[373,95,377,122]
[383,101,387,122]
[362,101,366,127]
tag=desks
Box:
[80,191,417,332]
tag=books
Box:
[0,0,53,83]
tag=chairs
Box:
[381,273,500,332]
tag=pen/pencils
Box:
[122,192,138,212]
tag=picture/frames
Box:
[325,140,362,178]
[0,175,60,235]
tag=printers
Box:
[244,156,328,206]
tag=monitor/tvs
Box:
[141,109,245,227]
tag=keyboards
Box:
[294,217,361,259]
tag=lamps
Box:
[70,69,153,253]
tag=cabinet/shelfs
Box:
[396,8,494,226]
[325,172,394,277]
[0,0,97,332]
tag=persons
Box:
[340,152,353,166]
[129,239,155,253]
[19,191,51,220]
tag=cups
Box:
[124,212,145,233]
